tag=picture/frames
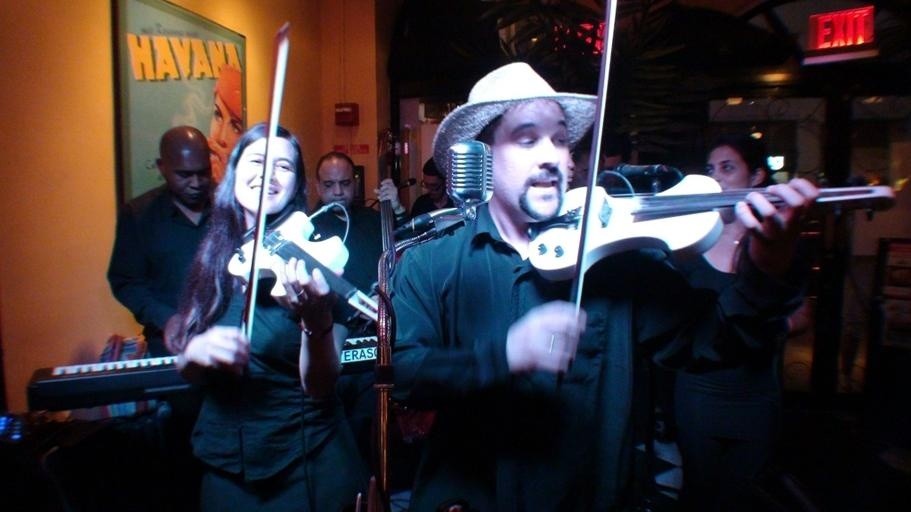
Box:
[113,0,248,206]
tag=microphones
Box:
[614,163,667,179]
[445,140,494,221]
[398,177,417,190]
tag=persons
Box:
[386,57,824,508]
[198,64,244,186]
[400,155,459,223]
[652,126,786,504]
[106,124,222,354]
[559,139,610,197]
[304,149,411,337]
[158,120,369,508]
[591,133,629,178]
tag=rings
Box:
[548,333,556,353]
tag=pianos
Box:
[26,336,380,412]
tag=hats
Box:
[429,60,600,176]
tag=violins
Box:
[528,172,896,280]
[227,210,379,321]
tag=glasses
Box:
[420,178,442,192]
